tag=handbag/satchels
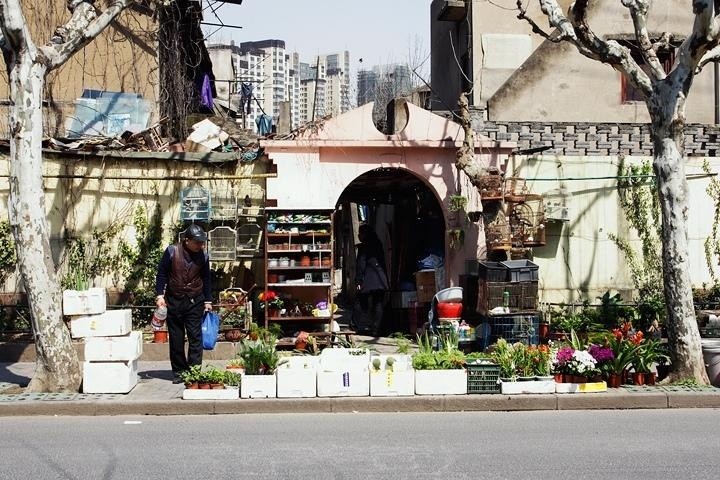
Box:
[200,309,219,350]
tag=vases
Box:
[268,309,278,317]
[152,330,168,344]
[267,242,331,283]
[295,342,306,349]
[500,372,656,389]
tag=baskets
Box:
[465,357,500,393]
[476,258,542,352]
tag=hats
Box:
[184,225,210,242]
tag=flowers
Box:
[258,291,284,310]
[490,321,672,378]
[296,331,309,344]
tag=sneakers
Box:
[172,375,186,384]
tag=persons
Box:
[153,224,214,385]
[346,221,390,339]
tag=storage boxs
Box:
[276,347,370,398]
[390,271,436,308]
[62,287,143,393]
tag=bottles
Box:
[503,291,510,307]
[459,320,476,340]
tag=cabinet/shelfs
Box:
[263,207,334,349]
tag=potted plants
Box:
[370,324,467,396]
[237,329,280,398]
[223,307,247,342]
[176,365,240,389]
[539,301,587,347]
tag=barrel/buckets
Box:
[151,302,168,330]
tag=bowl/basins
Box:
[435,285,464,318]
